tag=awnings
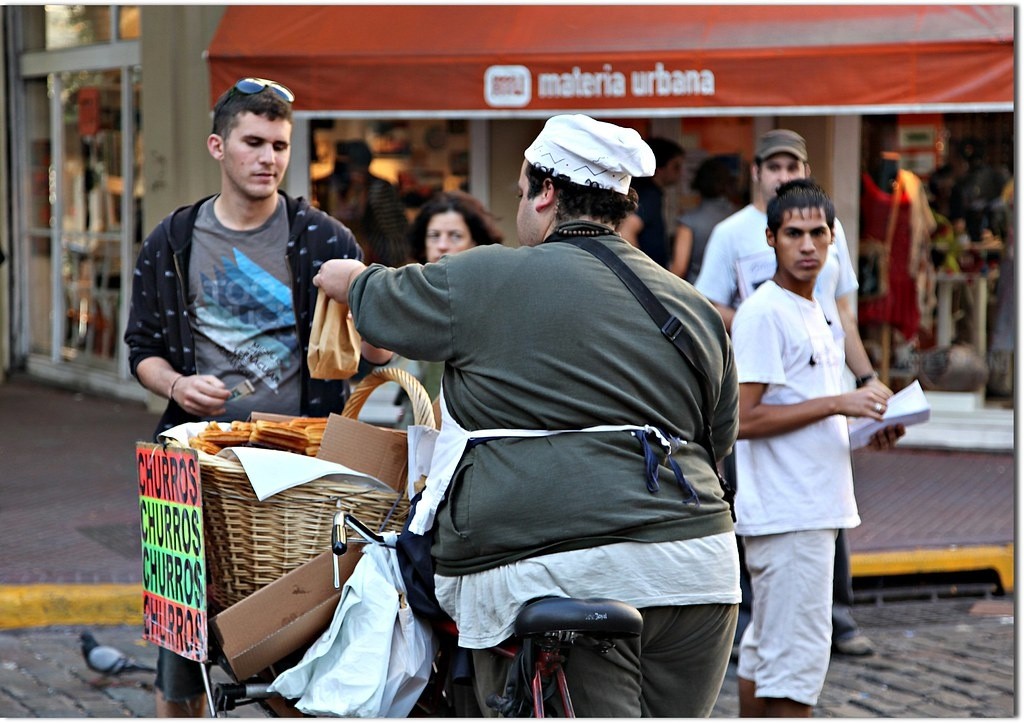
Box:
[203,7,1016,118]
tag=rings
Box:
[875,404,882,412]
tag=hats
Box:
[752,129,808,160]
[523,114,657,195]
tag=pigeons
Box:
[74,629,158,686]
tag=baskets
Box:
[164,367,435,609]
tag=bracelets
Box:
[169,376,181,399]
[855,370,878,388]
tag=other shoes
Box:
[832,630,873,656]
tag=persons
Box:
[344,139,411,268]
[310,113,742,718]
[615,135,684,266]
[859,152,937,361]
[408,192,503,404]
[728,179,905,718]
[694,129,894,666]
[123,78,404,718]
[669,153,750,285]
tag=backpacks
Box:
[857,239,888,299]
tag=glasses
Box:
[222,75,296,103]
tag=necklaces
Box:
[550,226,614,237]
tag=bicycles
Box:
[214,510,644,718]
